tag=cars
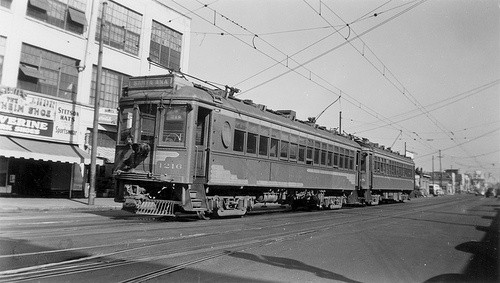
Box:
[467,178,500,199]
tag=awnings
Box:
[0,135,105,166]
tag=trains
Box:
[108,72,417,223]
